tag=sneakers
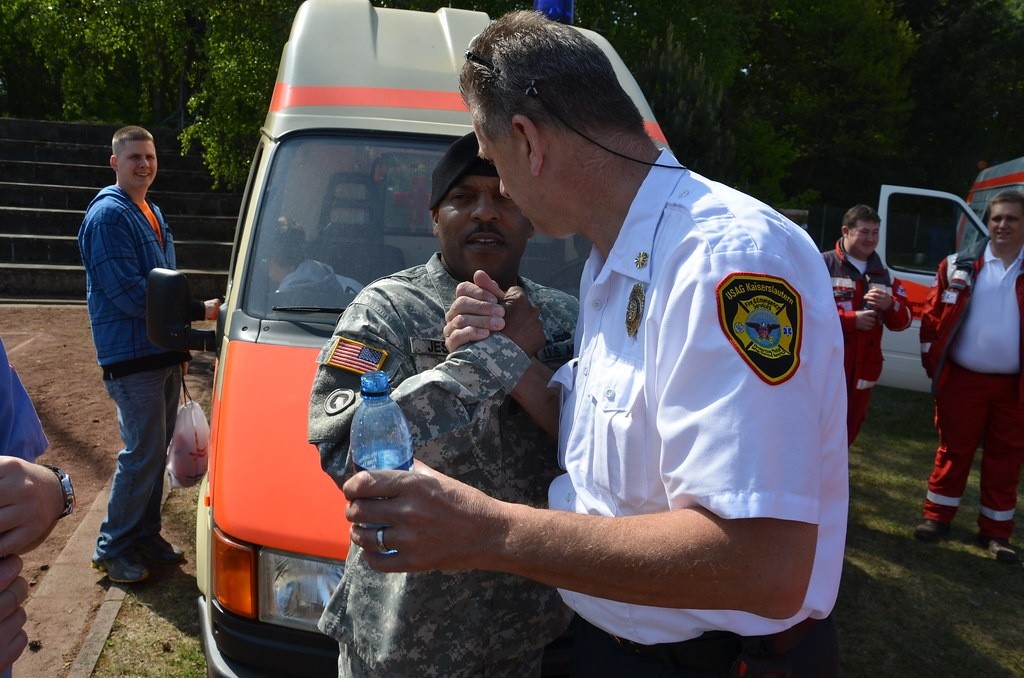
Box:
[916,520,952,539]
[92,551,150,583]
[131,532,184,564]
[983,535,1016,562]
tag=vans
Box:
[871,155,1024,394]
[144,0,676,678]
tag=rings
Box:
[375,528,388,551]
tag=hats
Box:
[429,132,499,210]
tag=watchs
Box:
[44,463,75,519]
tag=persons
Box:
[812,203,914,455]
[305,126,585,678]
[76,124,222,583]
[918,189,1024,562]
[341,10,851,678]
[1,337,78,678]
[268,217,365,309]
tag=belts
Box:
[604,618,820,673]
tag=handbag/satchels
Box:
[166,375,210,488]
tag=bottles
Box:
[351,370,414,556]
